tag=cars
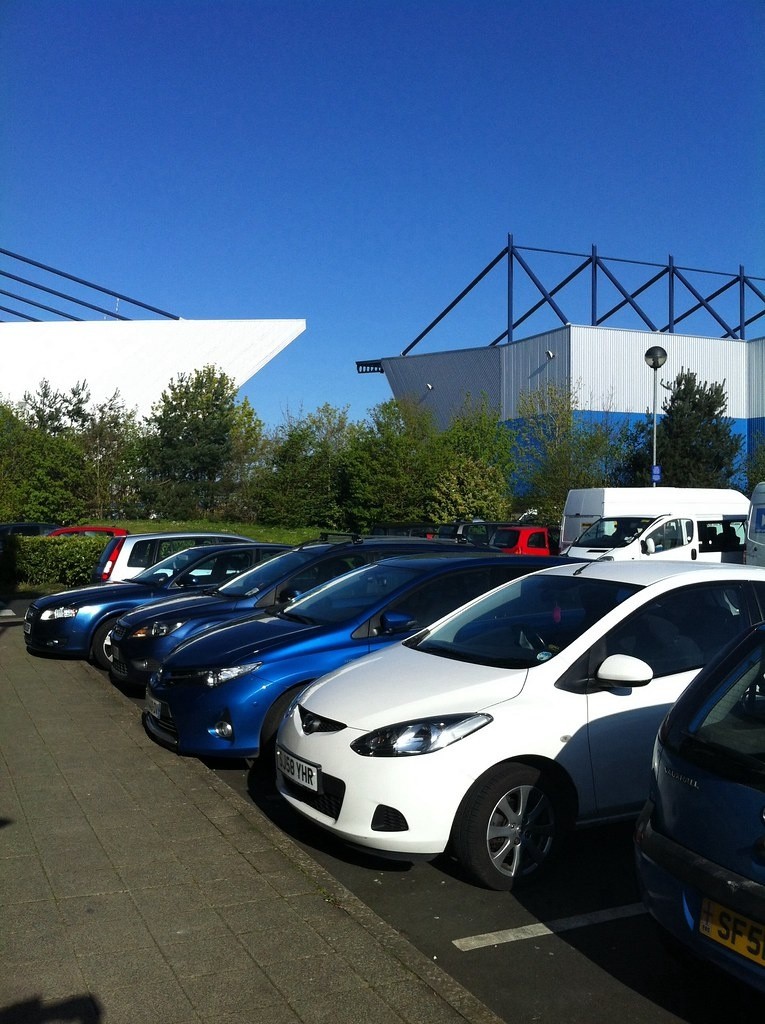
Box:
[46,526,128,542]
[275,560,765,888]
[635,621,765,993]
[24,542,294,671]
[0,523,59,538]
[142,552,605,759]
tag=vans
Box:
[92,534,255,585]
[561,513,749,565]
[560,487,753,555]
[745,482,765,566]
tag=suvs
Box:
[111,531,503,685]
[369,523,557,556]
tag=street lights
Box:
[644,346,668,486]
